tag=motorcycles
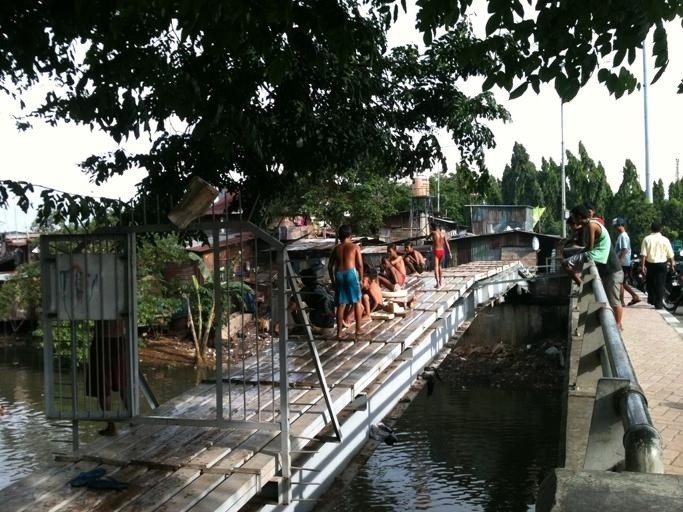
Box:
[624,250,682,314]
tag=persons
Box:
[402,240,425,278]
[83,316,139,437]
[326,224,365,337]
[557,201,628,334]
[271,269,335,338]
[610,217,642,307]
[424,220,451,288]
[639,221,676,309]
[335,243,408,326]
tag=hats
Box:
[612,218,626,227]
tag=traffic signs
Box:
[670,239,682,256]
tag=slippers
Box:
[627,299,641,306]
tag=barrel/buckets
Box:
[414,176,429,196]
